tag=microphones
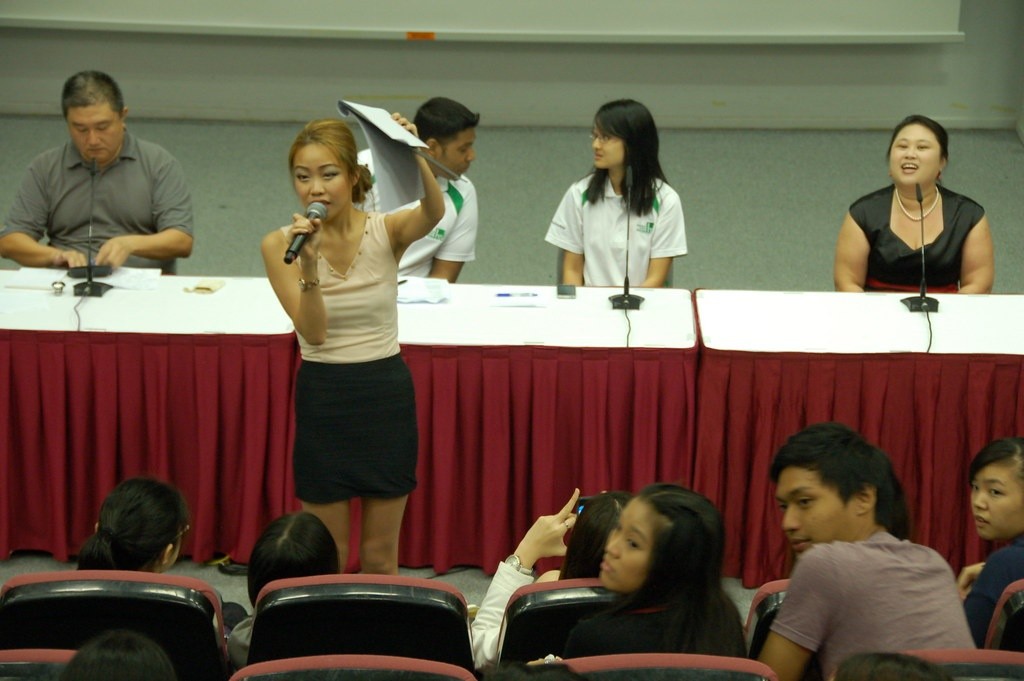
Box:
[899,183,939,312]
[283,202,327,264]
[609,165,645,310]
[74,157,114,297]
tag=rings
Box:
[564,522,570,529]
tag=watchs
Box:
[297,278,319,293]
[504,554,532,574]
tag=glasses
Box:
[590,132,617,144]
[174,523,190,541]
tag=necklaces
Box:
[894,183,939,220]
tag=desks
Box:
[0,270,700,577]
[692,288,1023,588]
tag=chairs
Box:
[1,571,1023,680]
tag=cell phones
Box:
[577,496,591,518]
[557,284,576,298]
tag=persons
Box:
[53,422,1024,681]
[542,99,688,287]
[0,70,194,275]
[351,95,480,284]
[262,109,447,577]
[835,113,995,293]
